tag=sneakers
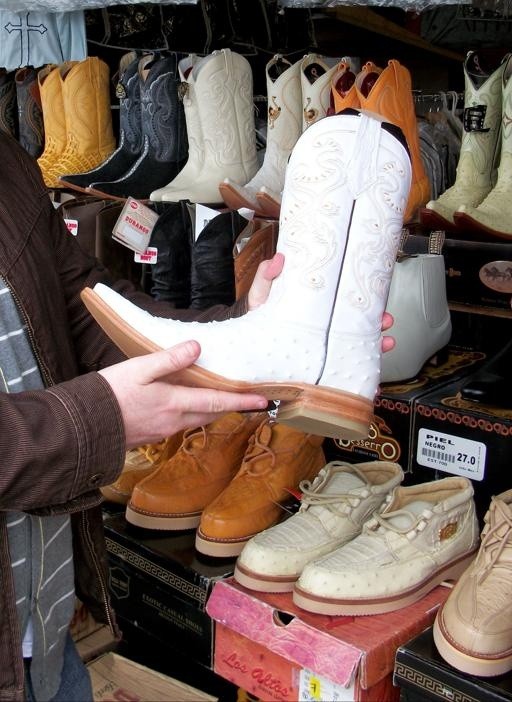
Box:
[196,418,325,557]
[100,425,211,502]
[433,488,512,677]
[293,477,480,615]
[234,461,403,593]
[126,412,270,531]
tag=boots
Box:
[379,254,453,386]
[0,1,512,242]
[78,109,412,443]
[51,198,277,307]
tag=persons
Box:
[1,128,398,700]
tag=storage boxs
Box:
[322,348,490,479]
[394,626,511,702]
[409,346,511,496]
[101,510,235,670]
[208,567,450,702]
